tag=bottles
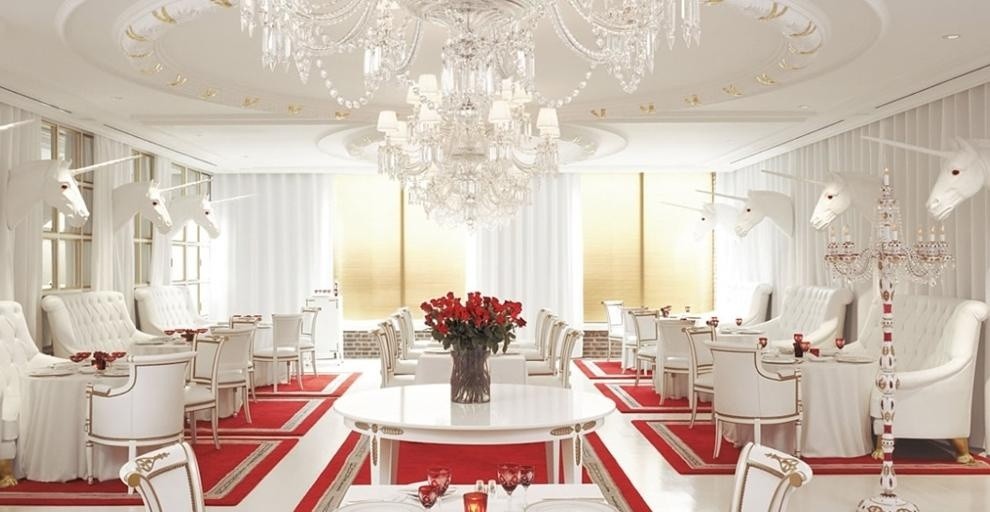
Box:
[476,479,498,504]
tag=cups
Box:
[463,491,489,511]
[425,469,453,495]
[758,337,769,349]
[418,484,438,510]
[659,304,743,333]
[792,333,820,358]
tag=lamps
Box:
[227,1,701,227]
[823,165,958,512]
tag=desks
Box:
[330,378,617,484]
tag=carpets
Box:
[293,429,650,512]
[246,370,363,399]
[0,433,303,509]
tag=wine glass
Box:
[835,337,846,355]
[67,349,128,374]
[495,461,522,512]
[519,465,535,509]
[164,327,208,343]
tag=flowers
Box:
[419,290,527,396]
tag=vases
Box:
[447,347,493,404]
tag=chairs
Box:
[374,288,581,387]
[600,278,990,467]
[117,440,207,512]
[730,439,816,511]
[0,282,341,487]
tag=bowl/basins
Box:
[779,346,795,354]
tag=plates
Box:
[839,355,873,365]
[820,350,840,356]
[332,500,424,512]
[524,499,620,512]
[735,328,763,335]
[761,356,794,364]
